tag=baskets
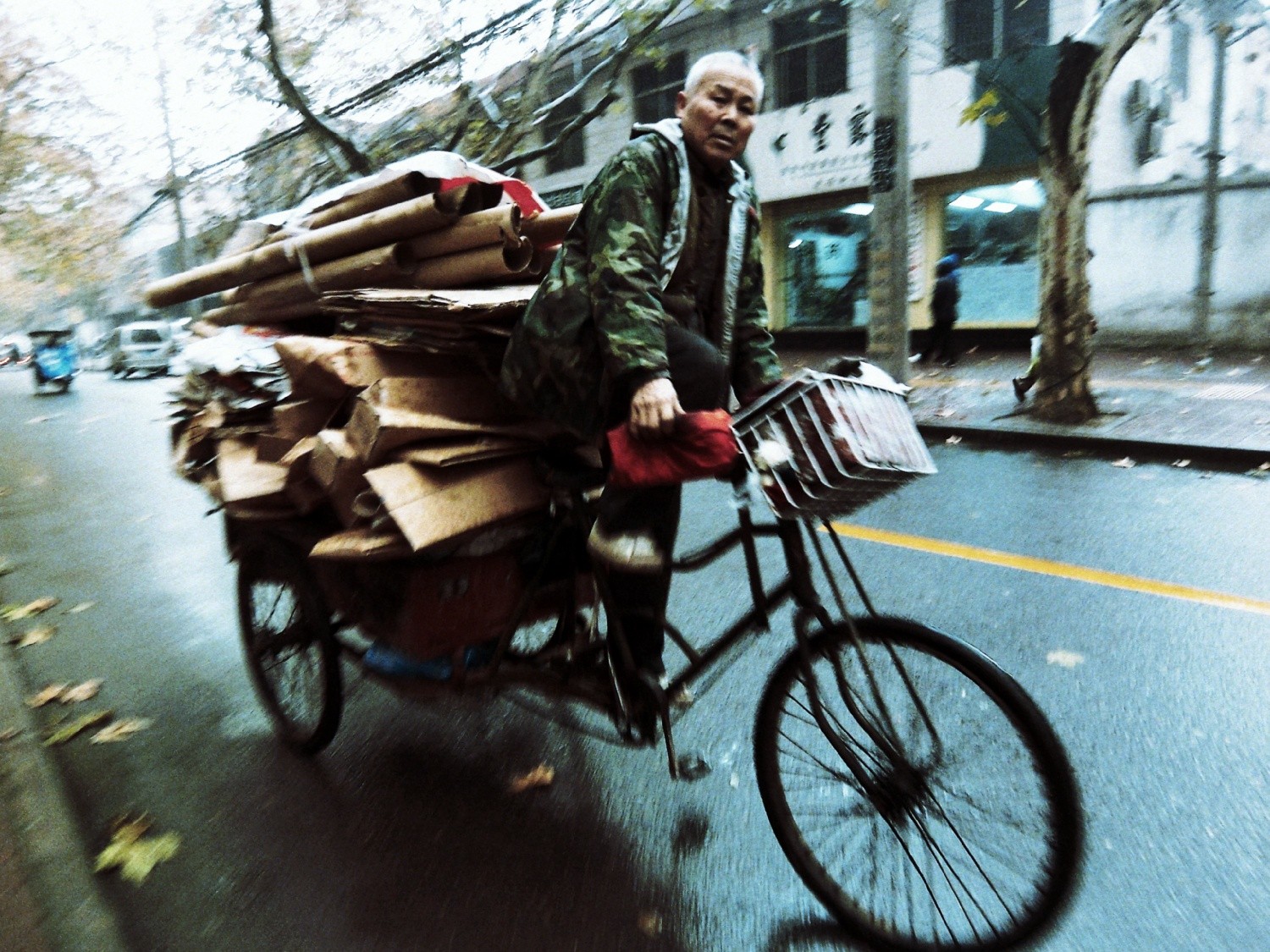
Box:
[726,367,938,525]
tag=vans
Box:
[110,320,172,380]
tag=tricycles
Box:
[23,326,79,393]
[224,367,1088,952]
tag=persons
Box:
[1012,247,1093,402]
[501,49,782,706]
[918,254,962,367]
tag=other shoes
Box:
[585,519,663,573]
